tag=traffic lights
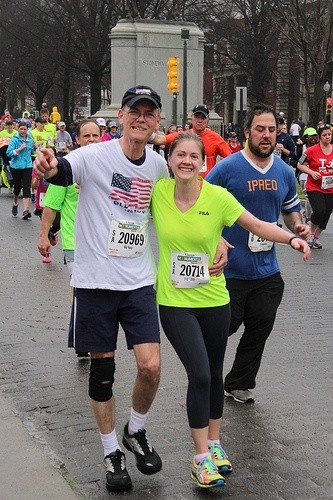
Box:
[167,58,179,94]
[325,97,332,115]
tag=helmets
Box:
[303,127,318,136]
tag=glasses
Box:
[170,128,176,132]
[122,107,160,121]
[320,124,330,128]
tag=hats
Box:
[121,85,162,111]
[96,118,106,126]
[7,104,66,132]
[193,104,209,117]
[229,131,238,138]
[108,122,116,128]
[177,125,183,128]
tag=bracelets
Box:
[289,235,303,249]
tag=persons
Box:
[6,122,38,220]
[297,123,333,249]
[76,131,311,488]
[0,103,124,203]
[146,112,333,228]
[37,118,102,357]
[31,146,61,263]
[203,102,310,404]
[149,103,233,179]
[33,87,228,493]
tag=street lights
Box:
[180,27,190,131]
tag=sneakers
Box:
[50,231,58,246]
[12,203,18,217]
[102,449,132,492]
[224,385,257,404]
[190,456,227,488]
[9,187,14,193]
[31,194,35,202]
[313,236,322,249]
[23,209,31,219]
[307,234,315,247]
[122,421,163,476]
[207,442,233,472]
[42,257,51,263]
